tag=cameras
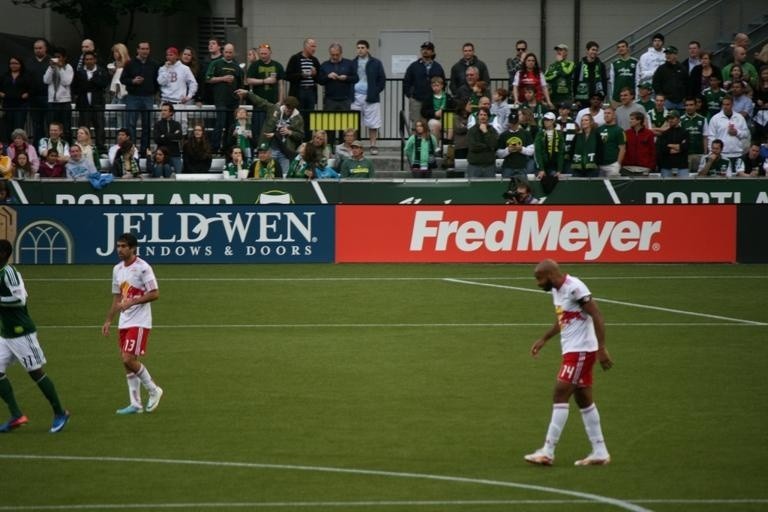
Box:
[503,191,520,201]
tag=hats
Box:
[256,141,273,151]
[555,43,568,51]
[663,45,678,55]
[508,100,572,124]
[664,110,680,120]
[166,47,179,56]
[419,41,436,53]
[350,140,363,149]
[636,81,654,91]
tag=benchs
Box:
[68,101,254,151]
[96,155,340,182]
[432,102,526,177]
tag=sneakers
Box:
[369,146,378,155]
[145,385,164,413]
[0,415,31,433]
[116,402,144,413]
[48,410,72,433]
[574,453,610,466]
[523,448,554,466]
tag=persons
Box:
[522,259,614,467]
[0,239,70,434]
[101,232,164,414]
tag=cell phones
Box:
[512,144,516,146]
[51,58,59,62]
[271,73,276,78]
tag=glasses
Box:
[259,43,272,53]
